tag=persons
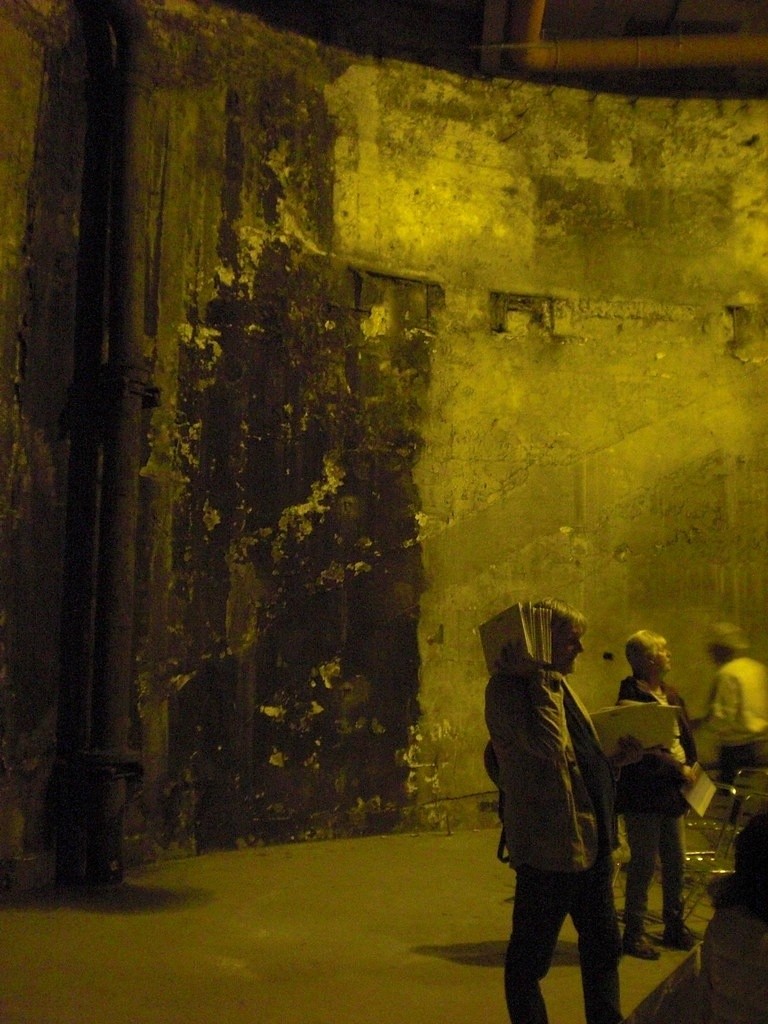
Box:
[477,598,679,1022]
[618,811,768,1023]
[690,622,768,793]
[612,630,717,957]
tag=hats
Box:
[711,621,751,650]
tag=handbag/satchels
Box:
[483,739,511,863]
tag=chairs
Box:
[609,761,768,939]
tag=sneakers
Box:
[663,928,695,951]
[622,934,661,960]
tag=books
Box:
[680,762,715,817]
[589,700,681,755]
[479,601,552,676]
